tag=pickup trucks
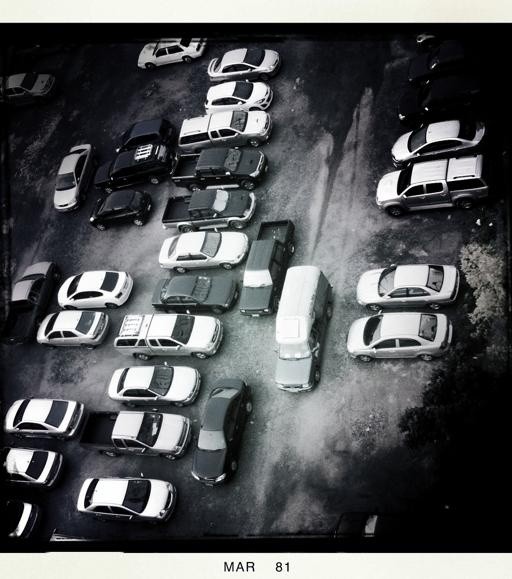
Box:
[161,146,269,233]
[238,218,295,317]
[79,408,193,460]
[3,261,61,345]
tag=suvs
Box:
[376,154,494,216]
[177,108,272,153]
[114,309,223,360]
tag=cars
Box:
[137,37,207,69]
[152,228,249,314]
[346,264,460,361]
[36,270,133,350]
[390,120,486,169]
[3,398,85,538]
[54,119,176,230]
[107,361,201,408]
[0,71,56,97]
[77,472,177,525]
[204,48,281,114]
[191,378,253,487]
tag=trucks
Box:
[276,266,333,393]
